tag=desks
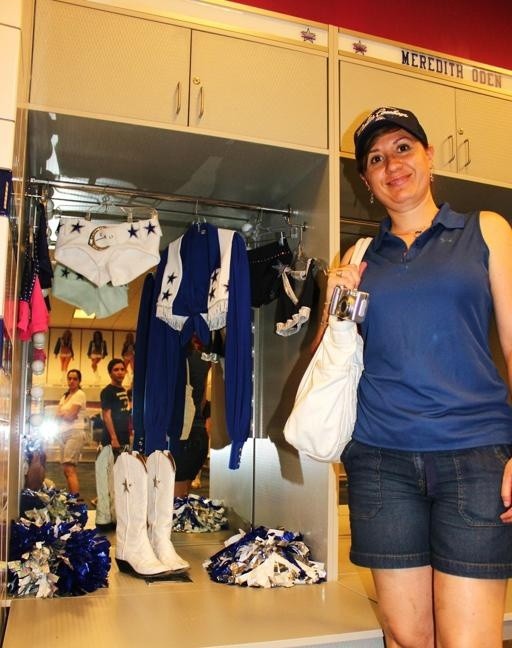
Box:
[338,565,512,644]
[1,573,387,648]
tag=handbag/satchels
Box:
[283,316,363,464]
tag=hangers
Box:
[55,185,158,226]
[190,194,324,278]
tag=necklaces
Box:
[390,229,423,237]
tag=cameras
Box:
[328,285,370,324]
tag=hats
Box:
[353,105,429,162]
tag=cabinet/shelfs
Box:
[333,47,512,202]
[17,0,332,157]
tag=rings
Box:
[336,270,343,277]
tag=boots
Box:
[95,446,117,532]
[113,451,191,580]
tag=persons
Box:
[310,106,512,648]
[53,331,74,372]
[90,359,129,506]
[122,333,135,370]
[87,331,108,372]
[55,370,87,498]
[25,413,46,492]
[169,397,209,496]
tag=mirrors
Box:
[15,177,255,553]
